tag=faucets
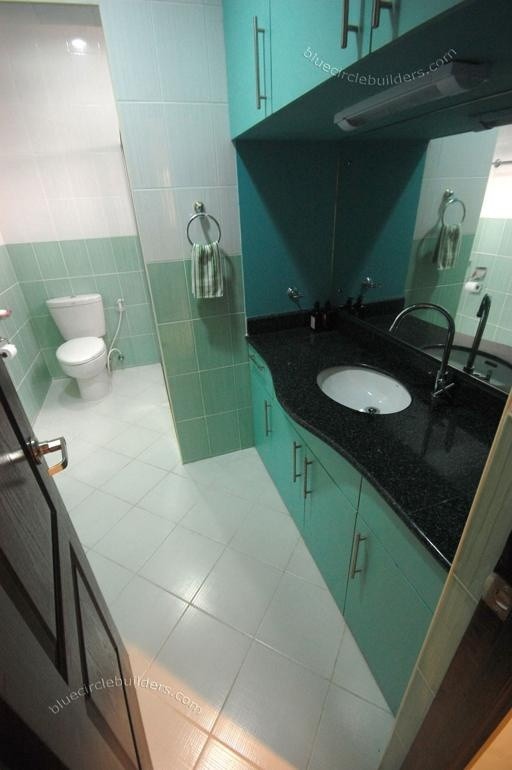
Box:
[388,303,456,396]
[463,294,491,373]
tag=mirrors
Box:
[323,85,510,406]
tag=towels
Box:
[432,224,461,270]
[191,242,225,299]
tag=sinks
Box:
[315,363,412,415]
[419,343,512,394]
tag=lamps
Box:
[332,59,472,132]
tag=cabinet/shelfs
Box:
[219,1,272,141]
[247,343,362,618]
[273,0,510,141]
[341,478,447,718]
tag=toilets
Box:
[44,292,112,402]
